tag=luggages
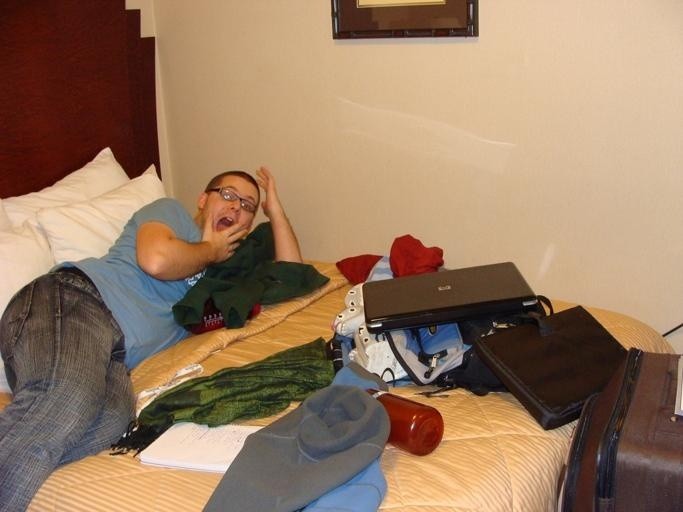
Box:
[553,347,682,512]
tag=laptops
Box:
[362,261,539,336]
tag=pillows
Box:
[26,162,168,259]
[0,146,132,238]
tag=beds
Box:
[0,258,677,511]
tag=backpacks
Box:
[328,288,554,398]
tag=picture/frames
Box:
[331,0,479,39]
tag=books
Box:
[138,422,264,474]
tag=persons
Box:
[1,167,302,510]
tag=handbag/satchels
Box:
[471,295,628,431]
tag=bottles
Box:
[364,387,445,458]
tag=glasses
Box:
[204,188,256,216]
